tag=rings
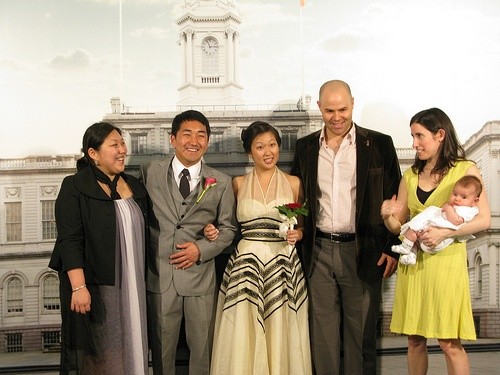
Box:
[186,260,190,263]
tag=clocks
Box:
[200,35,220,56]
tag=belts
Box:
[316,228,357,244]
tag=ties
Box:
[91,163,122,201]
[179,168,190,199]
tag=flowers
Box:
[273,202,309,231]
[196,176,218,203]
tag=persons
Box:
[391,175,482,265]
[204,121,313,375]
[138,110,237,375]
[50,123,149,375]
[292,80,403,375]
[382,107,492,375]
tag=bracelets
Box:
[72,285,86,293]
